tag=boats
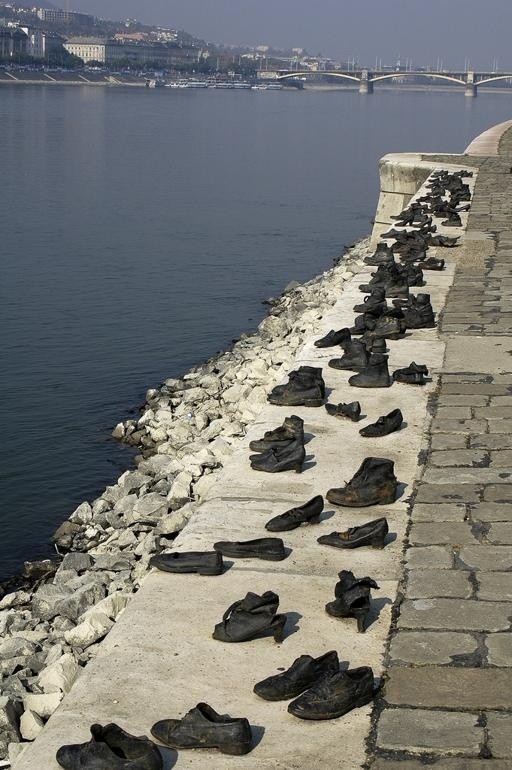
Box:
[168,77,284,90]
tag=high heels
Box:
[265,494,325,532]
[317,518,390,550]
[248,438,306,473]
[325,568,380,633]
[212,588,287,645]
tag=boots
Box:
[325,457,396,509]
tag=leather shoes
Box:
[150,701,252,756]
[56,722,163,770]
[272,366,325,394]
[314,265,436,389]
[363,170,474,266]
[148,551,225,577]
[267,371,322,408]
[359,408,404,438]
[252,649,340,700]
[213,537,286,563]
[287,666,376,721]
[325,400,361,422]
